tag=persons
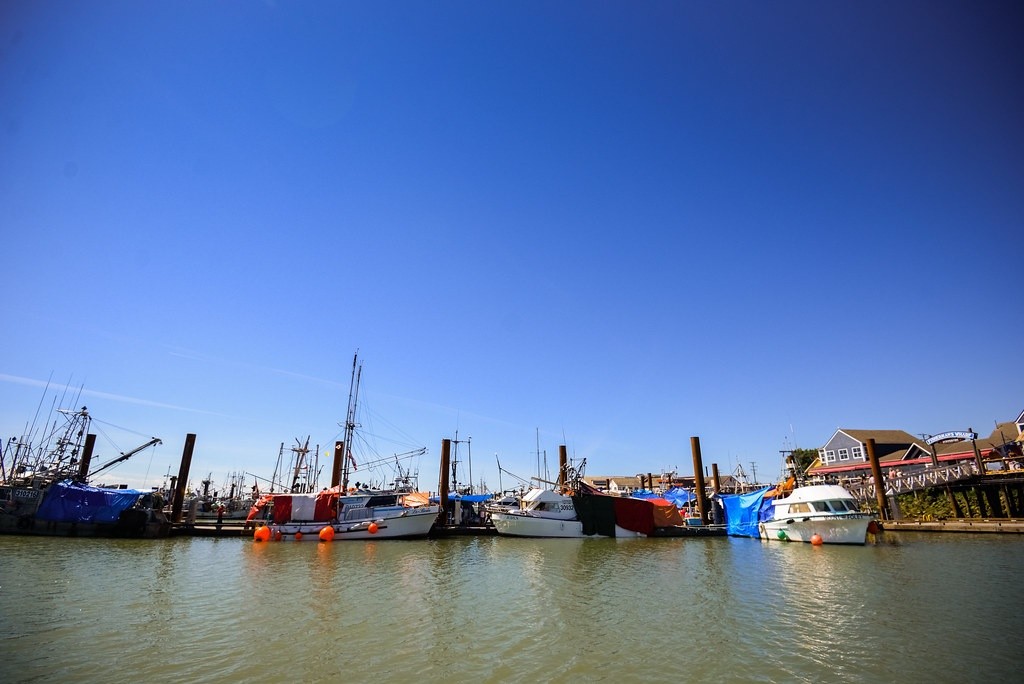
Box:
[217,505,225,524]
[798,467,907,501]
[251,486,262,499]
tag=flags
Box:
[349,450,357,471]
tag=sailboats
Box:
[0,348,712,540]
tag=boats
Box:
[576,474,732,539]
[488,457,656,541]
[758,483,870,547]
